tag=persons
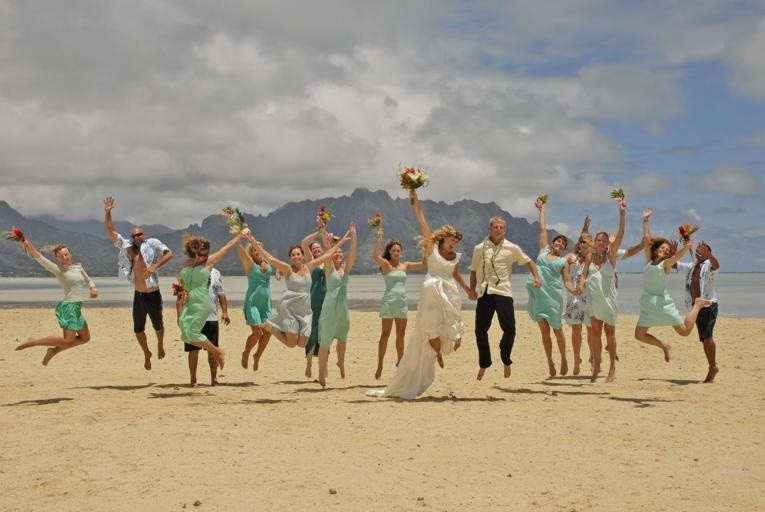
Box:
[374,228,427,379]
[102,195,174,368]
[669,239,720,383]
[13,240,98,367]
[172,213,358,389]
[467,215,542,381]
[524,205,580,377]
[565,199,645,384]
[365,190,478,399]
[634,207,712,362]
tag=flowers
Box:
[535,191,548,209]
[222,205,262,253]
[394,157,432,205]
[316,202,340,241]
[0,223,30,256]
[368,210,384,240]
[679,224,701,263]
[172,274,188,312]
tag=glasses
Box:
[131,233,143,238]
